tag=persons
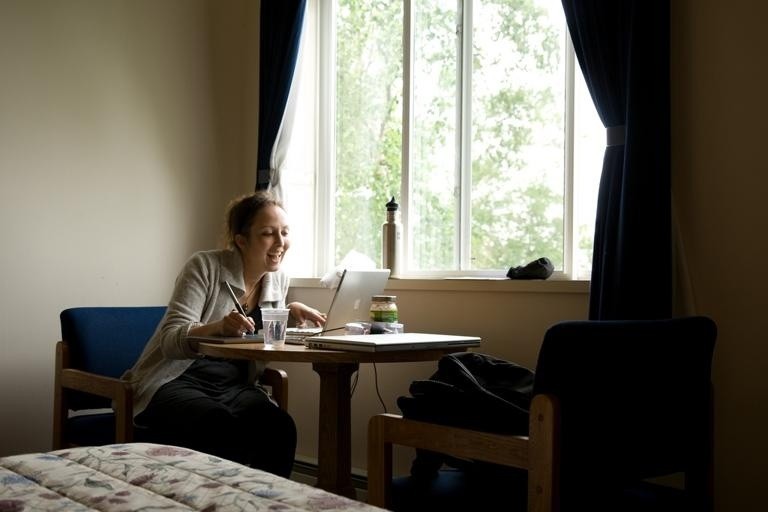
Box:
[112,191,327,479]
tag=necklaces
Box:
[243,283,259,311]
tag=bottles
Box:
[382,196,403,279]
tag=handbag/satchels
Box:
[396,352,535,436]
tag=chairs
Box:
[366,315,715,510]
[52,306,288,474]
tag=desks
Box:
[200,343,466,498]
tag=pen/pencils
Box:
[224,280,254,336]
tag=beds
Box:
[0,443,388,511]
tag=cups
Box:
[260,307,291,350]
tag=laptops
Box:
[305,332,482,353]
[258,268,392,345]
